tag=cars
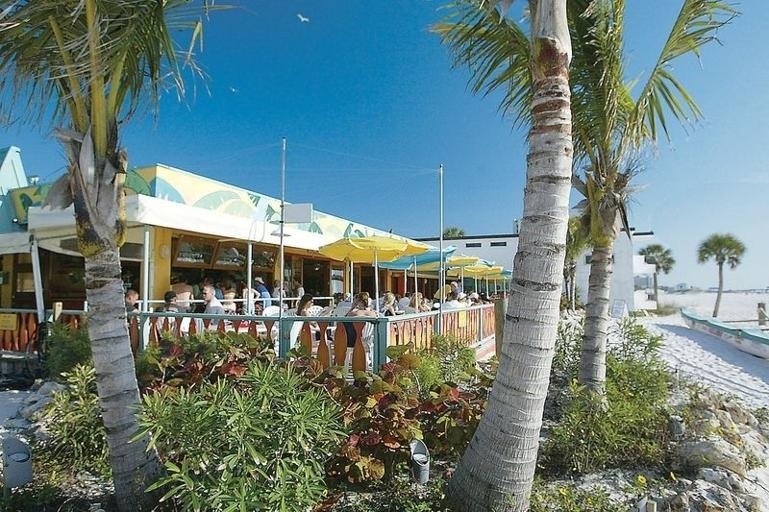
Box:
[645,288,652,294]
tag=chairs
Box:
[136,303,379,389]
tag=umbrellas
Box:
[324,236,513,309]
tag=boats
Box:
[680,307,769,360]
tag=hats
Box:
[451,281,479,300]
[254,277,265,284]
